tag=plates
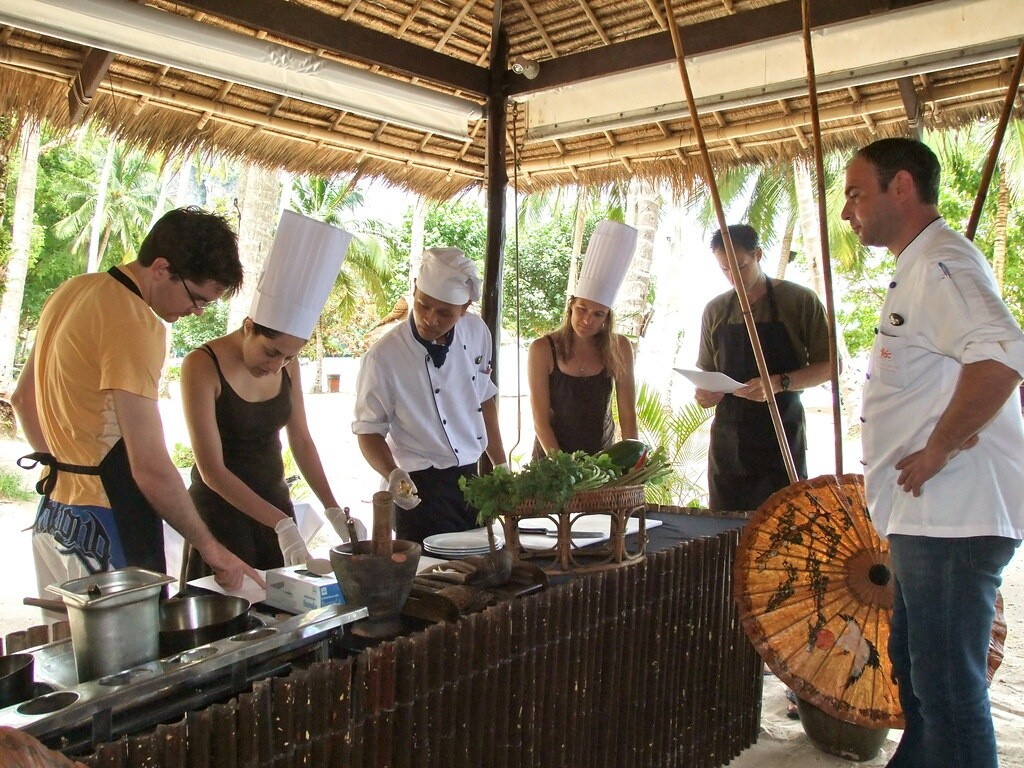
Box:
[422,532,504,560]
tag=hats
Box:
[415,247,481,305]
[573,220,638,309]
[248,209,352,340]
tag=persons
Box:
[693,223,837,513]
[841,138,1023,768]
[528,218,639,470]
[351,246,513,542]
[179,210,368,588]
[9,209,269,626]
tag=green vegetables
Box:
[458,448,614,526]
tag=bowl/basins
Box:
[0,653,35,708]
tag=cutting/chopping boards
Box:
[469,512,665,551]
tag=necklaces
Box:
[572,347,585,371]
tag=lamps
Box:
[512,57,540,80]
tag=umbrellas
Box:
[733,471,1007,727]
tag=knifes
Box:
[519,527,605,538]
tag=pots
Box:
[23,594,252,657]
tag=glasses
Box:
[168,259,213,309]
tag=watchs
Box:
[780,373,789,393]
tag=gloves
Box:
[324,507,367,543]
[387,468,421,510]
[274,516,312,567]
